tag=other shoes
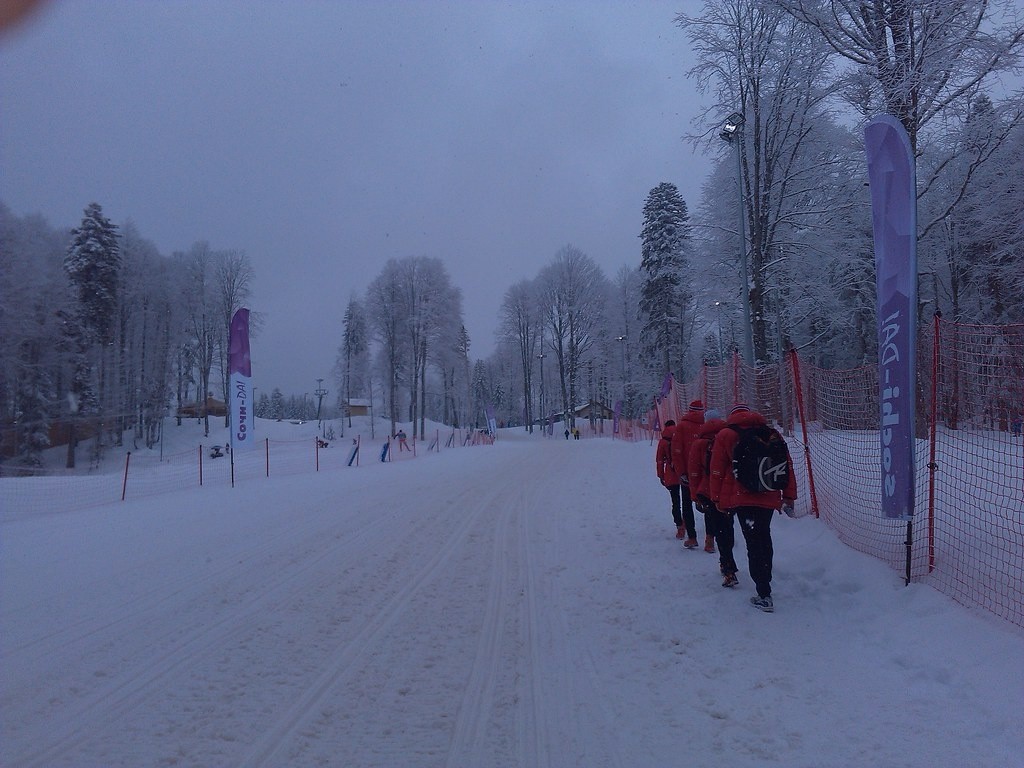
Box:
[676,528,685,540]
[681,517,686,528]
[684,537,698,546]
[704,536,715,552]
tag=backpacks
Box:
[662,436,673,471]
[726,422,790,496]
[699,435,714,478]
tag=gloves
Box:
[696,500,710,513]
[680,474,689,485]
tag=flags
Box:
[229,307,255,450]
[864,115,918,521]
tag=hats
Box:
[704,410,719,423]
[729,403,750,414]
[688,400,704,413]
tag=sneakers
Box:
[722,573,738,587]
[749,595,774,611]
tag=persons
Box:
[709,404,798,612]
[573,428,577,440]
[671,399,715,553]
[565,430,569,440]
[394,430,412,451]
[576,430,579,439]
[687,419,734,553]
[656,420,698,549]
